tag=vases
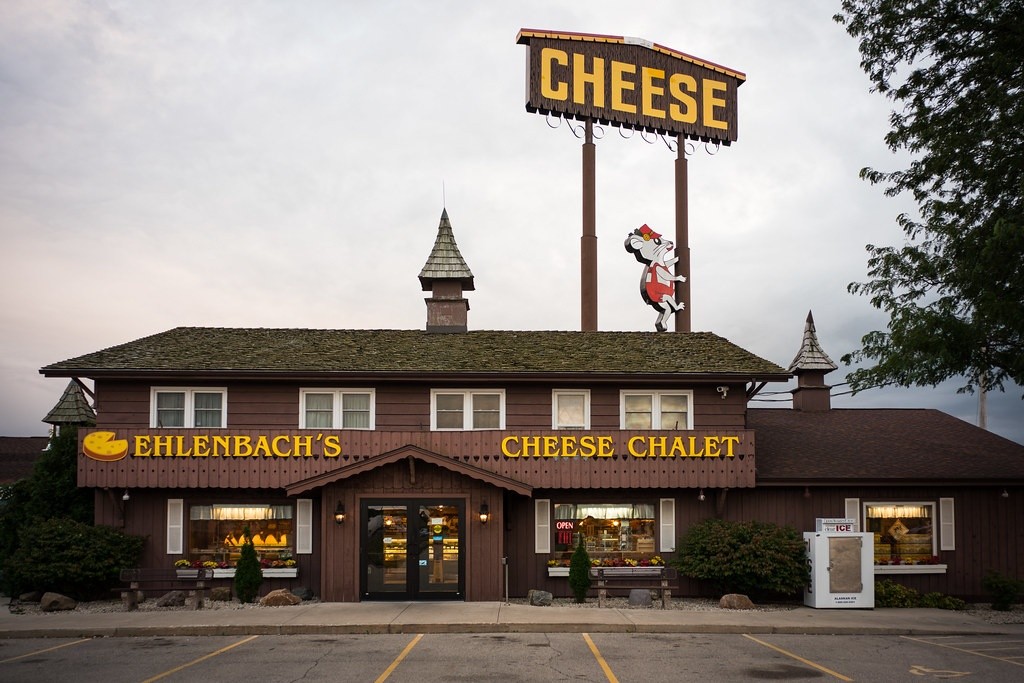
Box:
[179,565,294,569]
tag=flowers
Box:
[905,558,913,564]
[878,557,888,563]
[547,555,665,567]
[175,558,296,568]
[920,555,940,564]
[892,555,901,563]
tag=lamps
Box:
[334,500,345,524]
[478,500,489,524]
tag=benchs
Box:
[110,568,214,610]
[587,568,679,610]
